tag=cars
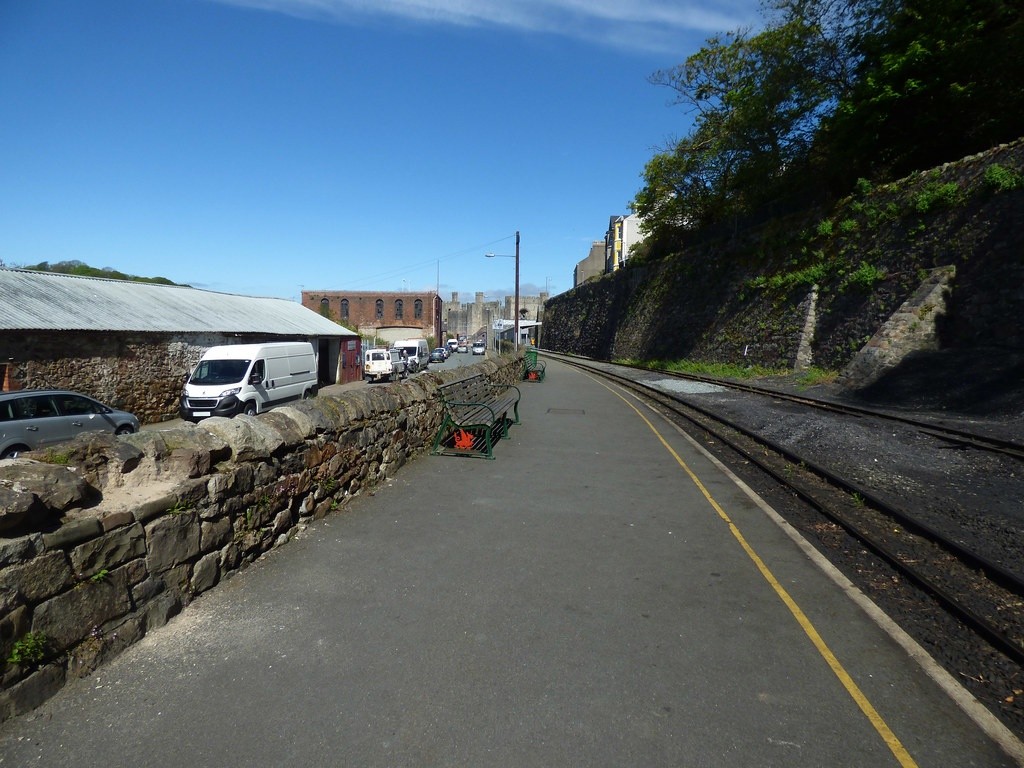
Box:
[434,345,453,359]
[458,346,468,353]
[429,349,445,363]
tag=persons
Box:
[402,350,410,375]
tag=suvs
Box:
[0,389,142,462]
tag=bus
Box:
[458,334,467,346]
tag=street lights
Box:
[484,252,520,350]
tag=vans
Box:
[179,342,320,425]
[394,339,430,373]
[471,341,486,355]
[365,348,410,382]
[446,338,459,351]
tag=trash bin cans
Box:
[526,350,538,366]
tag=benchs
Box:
[426,373,522,460]
[524,353,547,383]
